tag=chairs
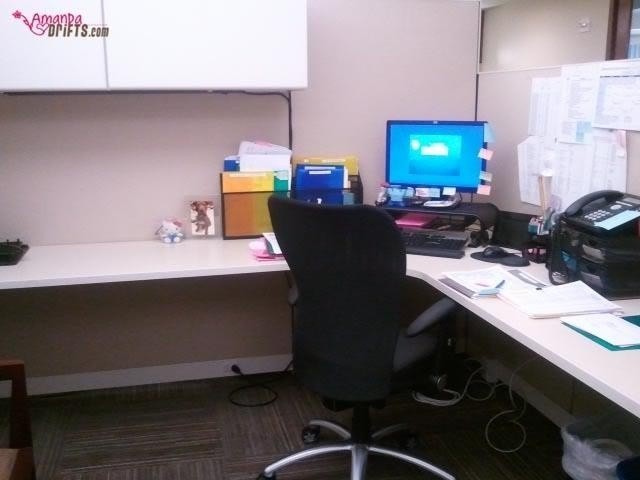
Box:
[257,193,465,478]
[0,358,37,480]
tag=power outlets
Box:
[225,360,241,372]
[479,354,499,384]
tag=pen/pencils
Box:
[495,280,505,288]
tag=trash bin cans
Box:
[560,406,640,480]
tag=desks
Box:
[1,240,640,432]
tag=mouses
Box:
[483,245,506,259]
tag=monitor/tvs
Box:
[384,118,486,208]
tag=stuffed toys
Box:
[158,219,184,243]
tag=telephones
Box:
[560,190,640,238]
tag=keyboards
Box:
[399,226,469,259]
